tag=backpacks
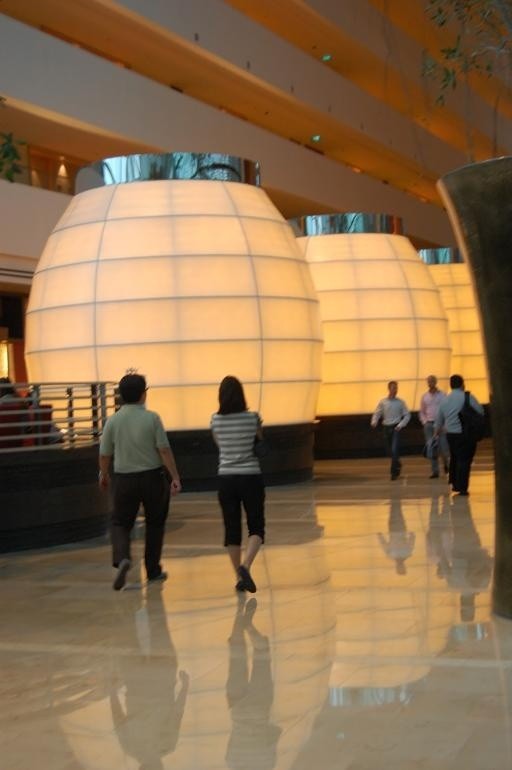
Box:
[454,391,490,442]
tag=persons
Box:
[369,380,412,481]
[97,373,182,591]
[209,375,271,592]
[431,375,484,494]
[416,375,448,478]
[1,377,27,449]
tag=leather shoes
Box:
[113,559,131,589]
[153,572,168,581]
[239,565,256,594]
[236,581,245,592]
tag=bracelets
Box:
[98,470,109,476]
